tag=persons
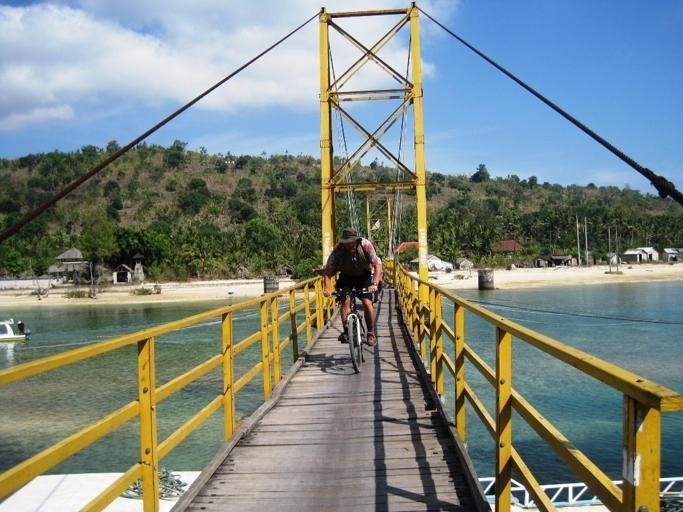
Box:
[368,245,384,304]
[16,320,24,335]
[323,229,382,346]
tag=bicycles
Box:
[324,289,377,375]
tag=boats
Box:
[0,317,33,344]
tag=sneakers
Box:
[338,333,349,341]
[367,332,376,346]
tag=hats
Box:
[339,229,362,244]
